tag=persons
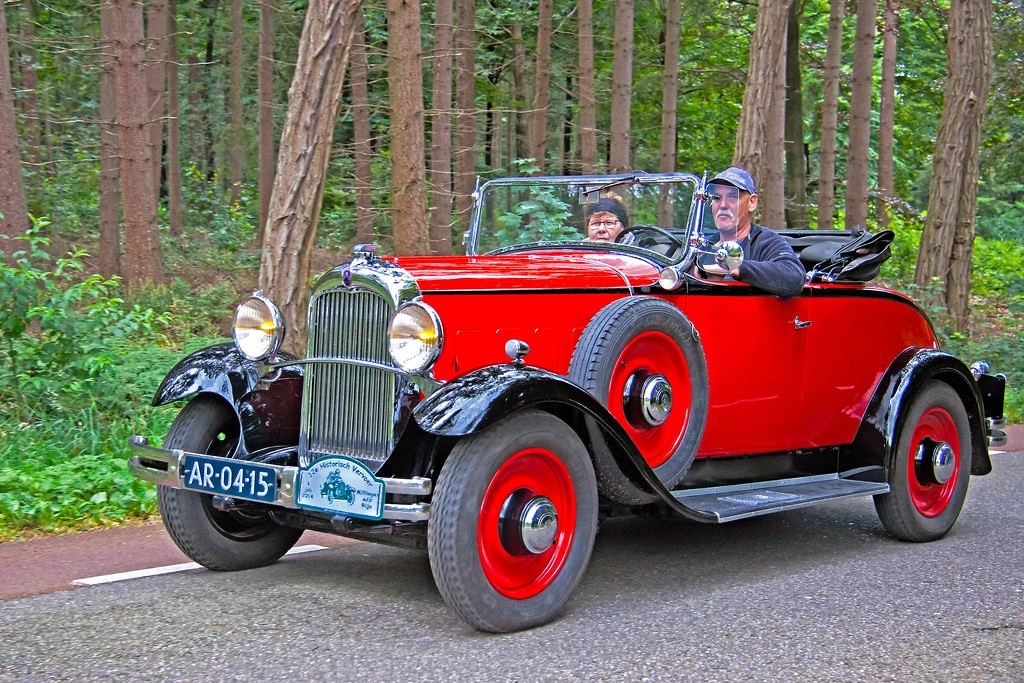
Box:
[693,167,807,301]
[584,192,644,247]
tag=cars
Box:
[128,171,1006,636]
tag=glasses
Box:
[588,219,620,229]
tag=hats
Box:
[706,167,755,195]
